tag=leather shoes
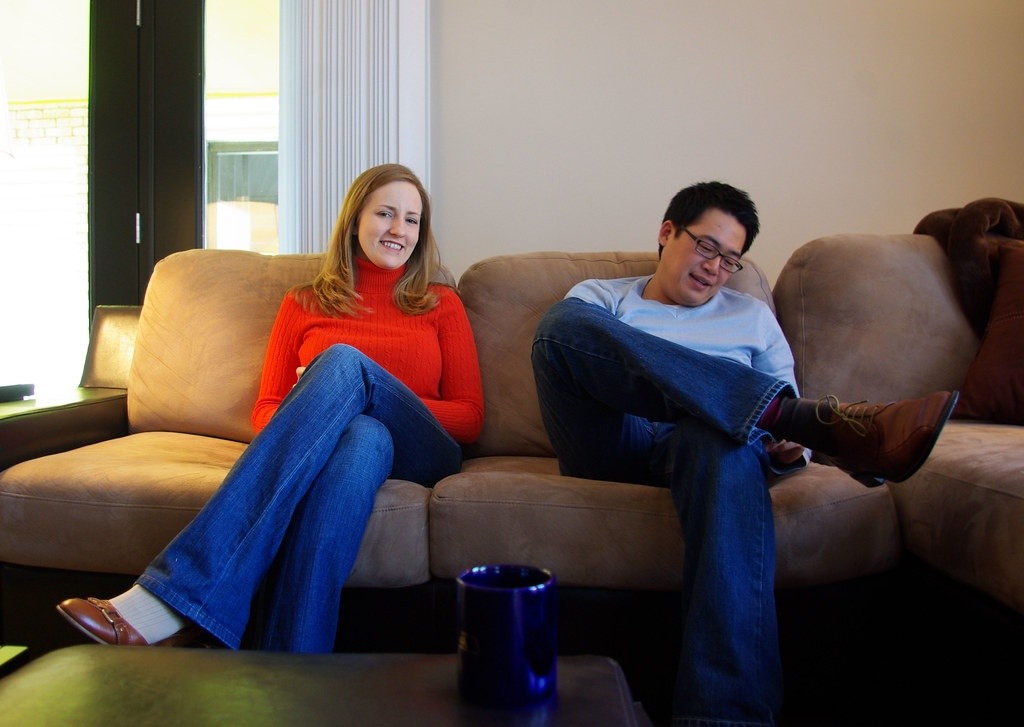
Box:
[808,391,959,488]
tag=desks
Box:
[0,643,638,727]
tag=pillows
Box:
[76,305,143,389]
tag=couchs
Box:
[0,233,1023,603]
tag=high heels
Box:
[56,581,219,648]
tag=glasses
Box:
[672,214,745,274]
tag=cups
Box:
[455,563,560,727]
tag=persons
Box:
[530,181,963,727]
[54,164,486,660]
